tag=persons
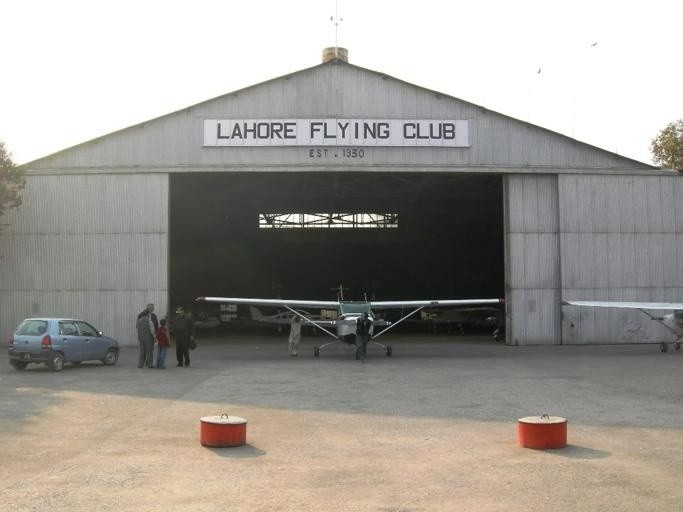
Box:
[286,313,305,358]
[354,311,371,363]
[135,303,159,365]
[135,309,157,368]
[170,304,193,368]
[155,319,169,370]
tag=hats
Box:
[176,306,184,314]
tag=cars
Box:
[9,317,118,371]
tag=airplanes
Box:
[555,298,681,352]
